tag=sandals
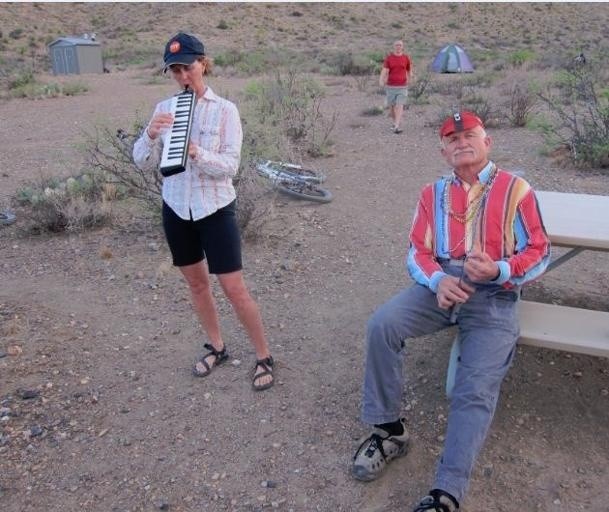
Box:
[192,343,229,378]
[253,355,275,390]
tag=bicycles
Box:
[249,156,332,203]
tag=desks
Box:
[445,190,609,400]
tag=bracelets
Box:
[147,127,156,139]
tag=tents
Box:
[429,42,475,75]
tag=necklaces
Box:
[439,163,498,262]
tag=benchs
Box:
[447,300,609,399]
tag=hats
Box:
[163,32,205,73]
[440,111,485,143]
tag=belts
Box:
[438,258,465,267]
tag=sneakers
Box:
[351,419,411,482]
[413,493,458,512]
[390,122,403,134]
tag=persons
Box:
[377,38,412,135]
[346,107,553,510]
[132,31,275,393]
